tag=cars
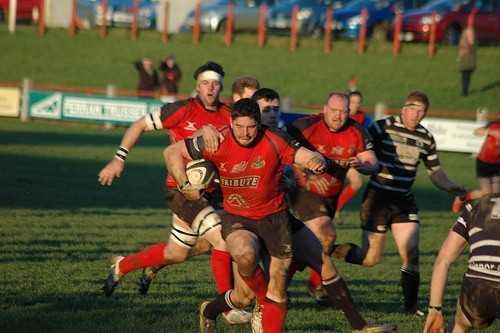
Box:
[319,0,398,42]
[391,0,500,46]
[183,0,264,35]
[93,1,157,31]
[0,0,42,23]
[264,0,337,40]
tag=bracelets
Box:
[429,305,443,312]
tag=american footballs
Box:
[177,159,218,193]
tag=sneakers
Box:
[101,254,123,296]
[406,305,429,317]
[252,300,265,333]
[199,302,217,333]
[222,308,253,325]
[452,194,465,212]
[351,321,399,333]
[138,265,159,297]
[305,283,334,307]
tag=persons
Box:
[459,28,476,96]
[423,192,500,333]
[97,62,398,333]
[452,120,500,212]
[330,92,467,317]
[135,55,181,99]
